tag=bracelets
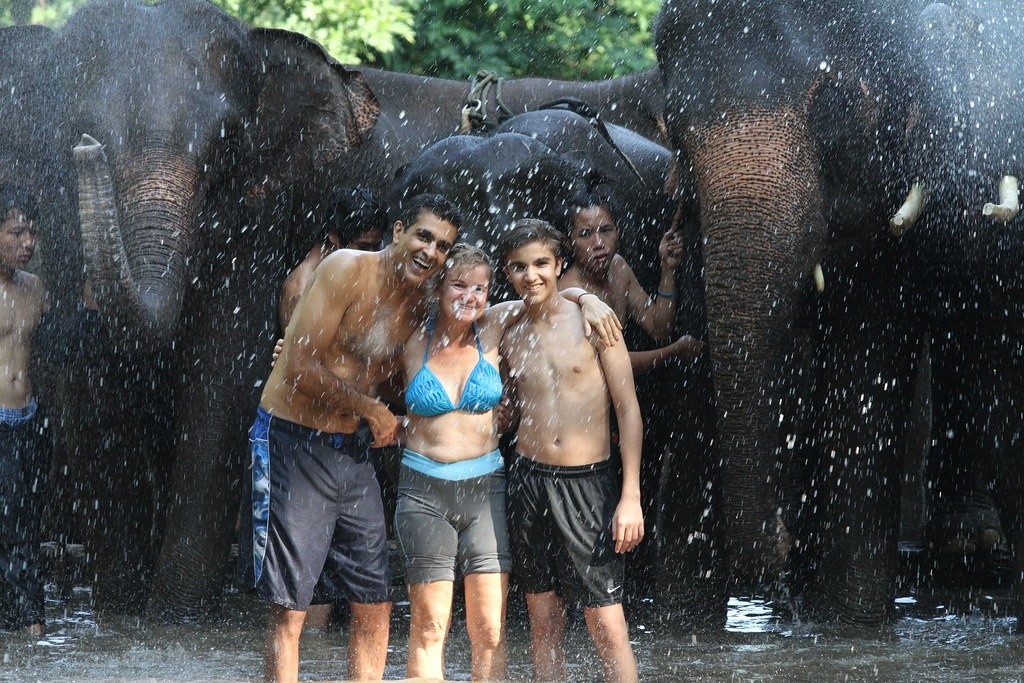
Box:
[656,285,680,298]
[577,291,596,302]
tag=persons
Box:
[392,218,644,682]
[553,197,704,375]
[277,188,387,637]
[0,180,54,640]
[234,193,514,681]
[271,194,622,682]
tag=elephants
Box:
[2,0,1022,631]
